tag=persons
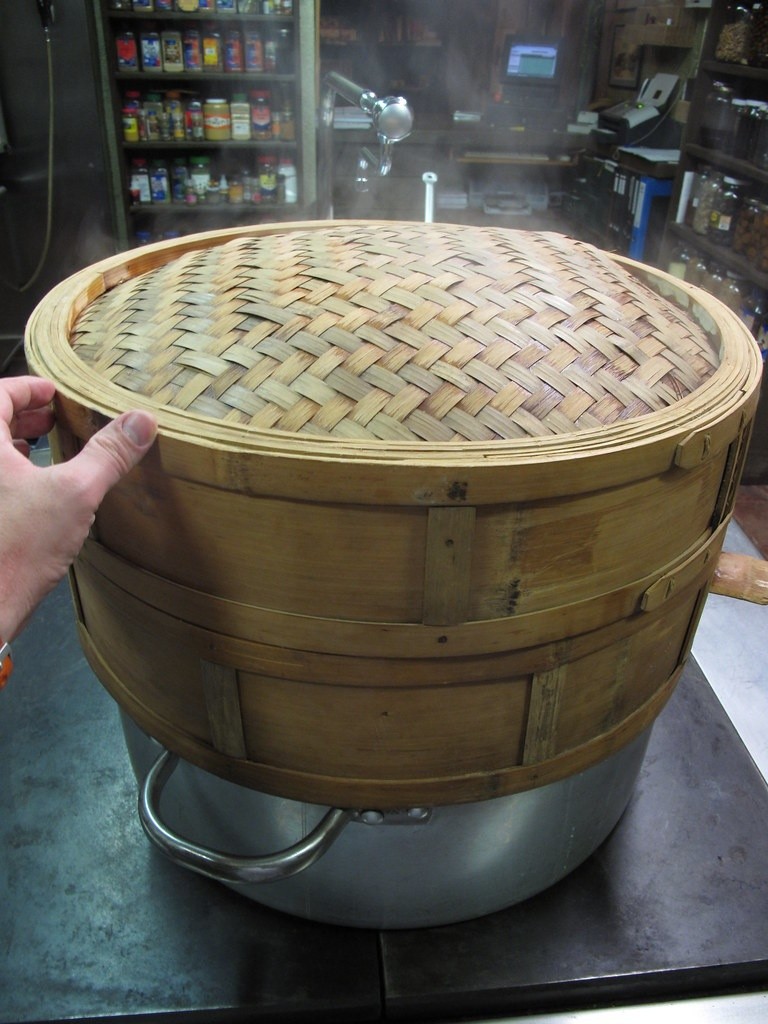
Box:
[0,376,158,674]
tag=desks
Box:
[318,108,599,219]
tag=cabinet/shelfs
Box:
[320,0,501,109]
[600,145,681,269]
[653,0,768,485]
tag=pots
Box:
[116,698,655,931]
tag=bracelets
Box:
[0,639,14,688]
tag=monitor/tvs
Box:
[498,34,569,85]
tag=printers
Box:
[592,72,682,147]
